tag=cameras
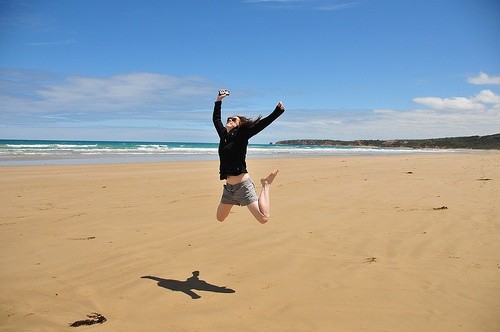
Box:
[219,89,230,95]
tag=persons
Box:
[213,89,286,224]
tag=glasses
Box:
[227,117,238,122]
